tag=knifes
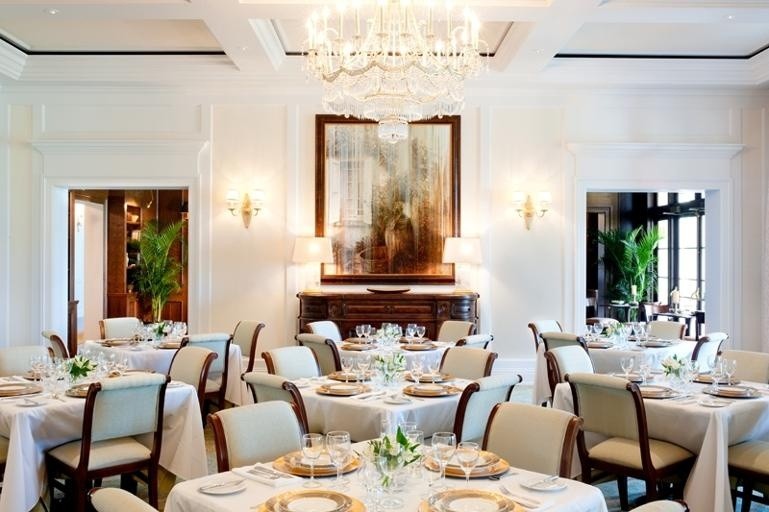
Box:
[528,475,559,488]
[255,465,293,479]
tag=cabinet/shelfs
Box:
[110,204,143,292]
[162,299,184,321]
[297,286,479,341]
[126,295,141,319]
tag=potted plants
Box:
[58,354,97,384]
[329,128,431,268]
[361,427,420,511]
[374,352,407,386]
[660,354,694,389]
[152,323,172,340]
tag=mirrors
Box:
[313,117,461,280]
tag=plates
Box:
[403,384,461,397]
[692,378,741,384]
[316,382,371,396]
[586,341,614,348]
[699,399,733,407]
[0,384,43,396]
[78,384,89,393]
[438,449,499,466]
[366,287,411,294]
[384,397,411,404]
[401,344,437,351]
[420,372,442,378]
[97,339,140,347]
[156,342,181,349]
[399,336,433,343]
[0,384,32,394]
[265,490,366,512]
[285,449,351,468]
[613,374,654,383]
[442,493,508,512]
[418,490,527,512]
[639,386,679,398]
[638,340,672,347]
[424,449,510,477]
[615,373,640,378]
[341,344,377,351]
[65,384,91,397]
[328,371,370,381]
[199,484,246,495]
[414,384,445,392]
[322,383,362,391]
[700,375,731,381]
[405,371,455,382]
[703,386,762,398]
[337,371,367,377]
[273,449,363,476]
[279,492,346,511]
[717,386,750,394]
[345,337,377,344]
[520,479,567,491]
[639,386,672,394]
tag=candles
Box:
[322,8,330,31]
[336,5,346,43]
[425,5,436,39]
[352,2,364,41]
[377,2,388,37]
[402,4,410,34]
[445,5,454,38]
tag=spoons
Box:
[248,469,281,479]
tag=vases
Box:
[357,246,390,271]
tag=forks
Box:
[499,485,541,504]
[200,478,244,490]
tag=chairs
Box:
[529,321,562,352]
[43,328,70,361]
[586,316,620,335]
[648,321,688,342]
[240,372,312,447]
[722,441,768,512]
[465,331,492,348]
[539,331,590,353]
[565,373,671,510]
[45,373,171,507]
[692,311,705,341]
[99,315,146,337]
[207,401,311,471]
[294,332,345,375]
[543,344,596,397]
[230,318,266,403]
[308,319,338,345]
[88,487,159,512]
[451,375,521,446]
[688,331,730,370]
[180,334,232,411]
[262,345,321,378]
[718,348,769,384]
[436,319,474,343]
[440,346,498,379]
[642,302,670,324]
[482,402,584,477]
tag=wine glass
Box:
[326,430,351,488]
[416,326,426,344]
[687,360,699,380]
[79,345,129,374]
[585,325,592,341]
[431,432,457,479]
[406,430,424,455]
[29,352,68,390]
[408,323,417,341]
[130,321,159,342]
[619,319,653,347]
[362,325,371,344]
[356,357,370,384]
[403,422,417,434]
[379,443,405,509]
[456,442,481,489]
[594,323,603,337]
[710,367,721,387]
[410,367,423,384]
[341,357,353,382]
[301,433,323,488]
[639,363,649,384]
[620,358,633,380]
[405,328,413,345]
[679,366,692,387]
[724,358,736,385]
[382,323,402,341]
[428,359,439,383]
[164,319,188,342]
[356,325,363,344]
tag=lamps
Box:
[292,236,334,292]
[251,186,265,218]
[535,193,549,221]
[442,236,484,295]
[225,186,241,219]
[511,188,527,222]
[301,3,490,145]
